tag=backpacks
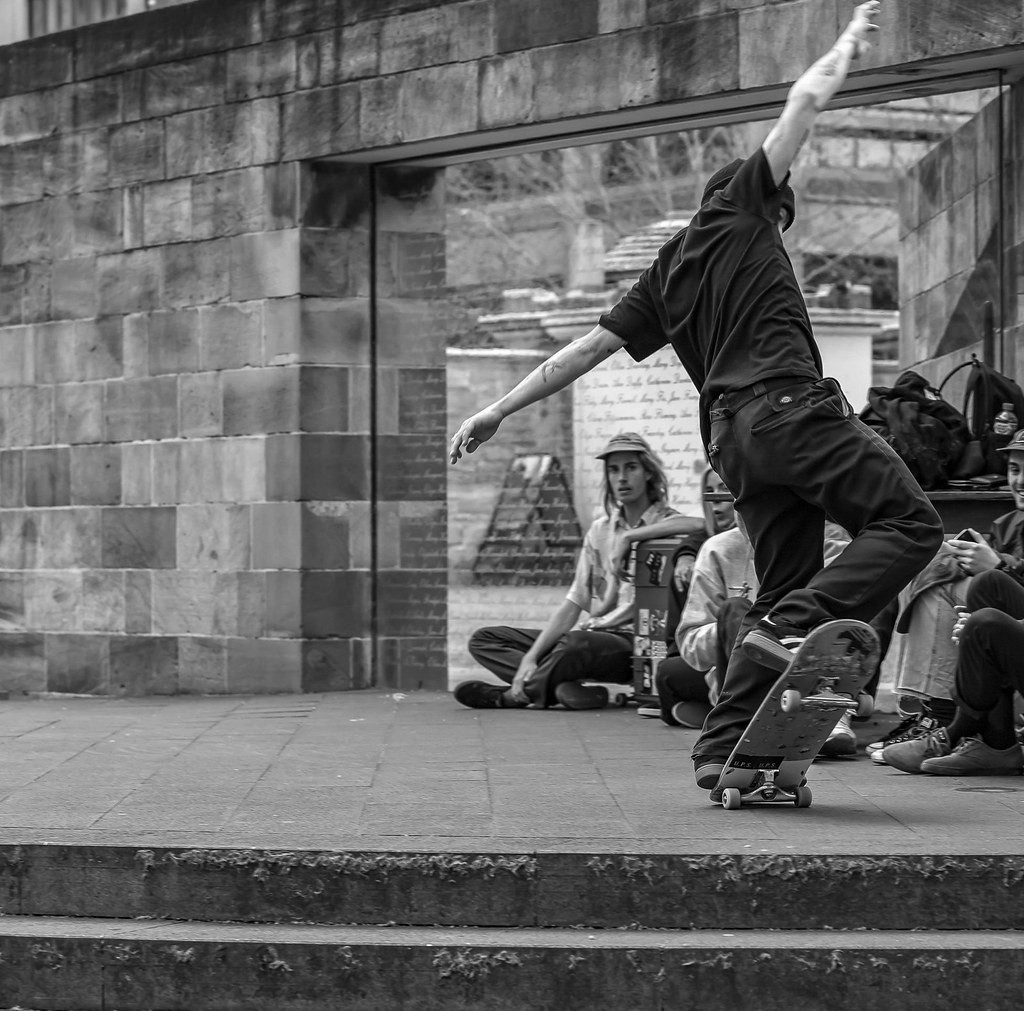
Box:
[938,352,1024,477]
[852,371,969,493]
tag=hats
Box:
[996,429,1024,451]
[595,432,648,459]
[700,159,795,231]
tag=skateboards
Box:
[710,619,880,810]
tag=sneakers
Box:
[919,737,1024,774]
[882,727,954,774]
[817,709,856,756]
[741,616,809,672]
[871,728,940,763]
[694,756,726,789]
[865,728,928,755]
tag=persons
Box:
[450,0,943,788]
[453,433,706,710]
[638,429,1024,777]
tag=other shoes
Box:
[556,683,609,711]
[637,702,662,717]
[454,680,512,708]
[672,701,714,728]
